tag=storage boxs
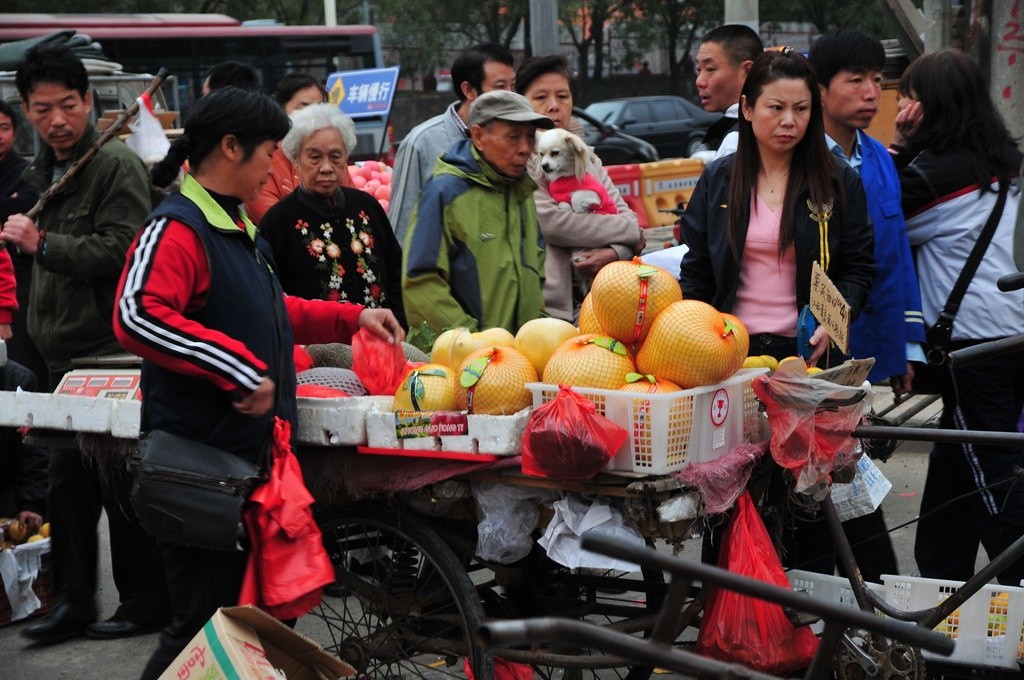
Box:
[0,365,774,488]
[784,570,1024,670]
[155,603,357,680]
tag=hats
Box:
[469,90,554,130]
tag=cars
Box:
[574,95,736,174]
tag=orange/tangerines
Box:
[742,354,823,374]
[1,521,50,548]
[933,594,1024,656]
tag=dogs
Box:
[526,128,630,262]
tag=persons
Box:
[679,51,898,585]
[0,46,165,638]
[689,23,764,165]
[257,103,410,339]
[517,55,640,328]
[386,42,516,250]
[202,61,259,96]
[400,90,546,338]
[111,88,295,680]
[891,50,1024,588]
[244,73,329,225]
[809,24,924,586]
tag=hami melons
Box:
[295,341,431,396]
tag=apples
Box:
[346,160,393,211]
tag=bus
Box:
[1,13,394,168]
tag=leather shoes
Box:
[20,601,98,642]
[85,604,162,639]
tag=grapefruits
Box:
[393,257,749,459]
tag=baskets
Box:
[783,567,949,647]
[522,367,771,480]
[880,574,1024,669]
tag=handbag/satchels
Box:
[697,490,818,666]
[130,430,260,552]
[911,324,952,395]
